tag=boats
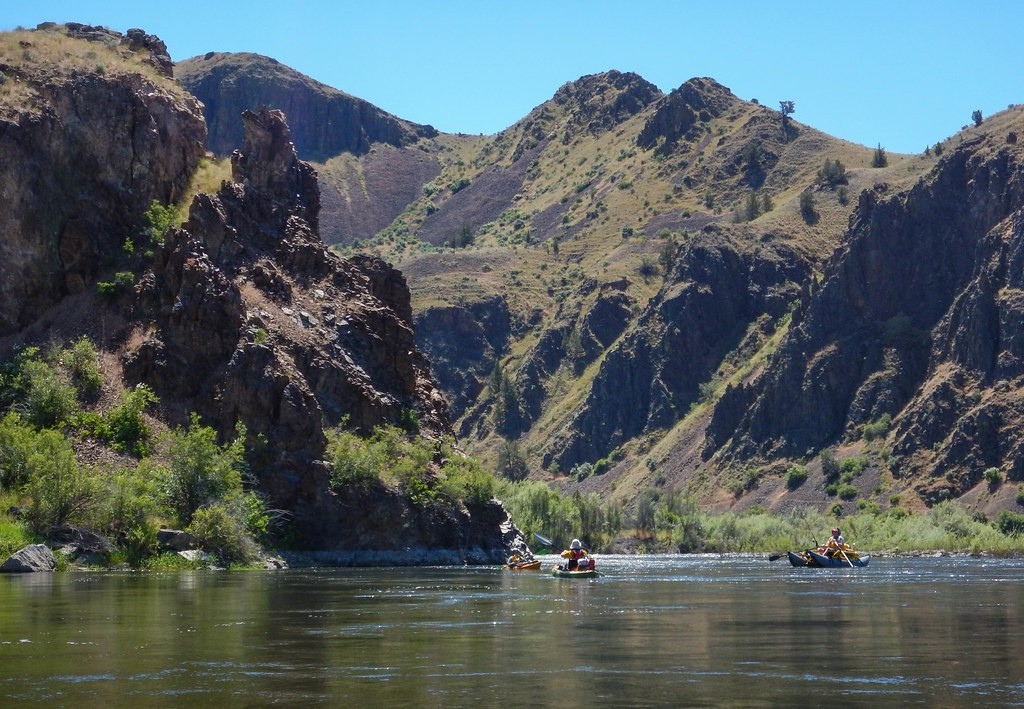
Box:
[550,562,601,579]
[503,561,542,572]
[787,550,815,567]
[806,548,871,568]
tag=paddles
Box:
[834,539,855,571]
[531,531,566,553]
[767,544,825,564]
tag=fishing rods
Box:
[792,500,821,548]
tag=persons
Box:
[560,538,588,571]
[825,528,845,548]
[507,550,528,567]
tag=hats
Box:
[570,539,582,549]
